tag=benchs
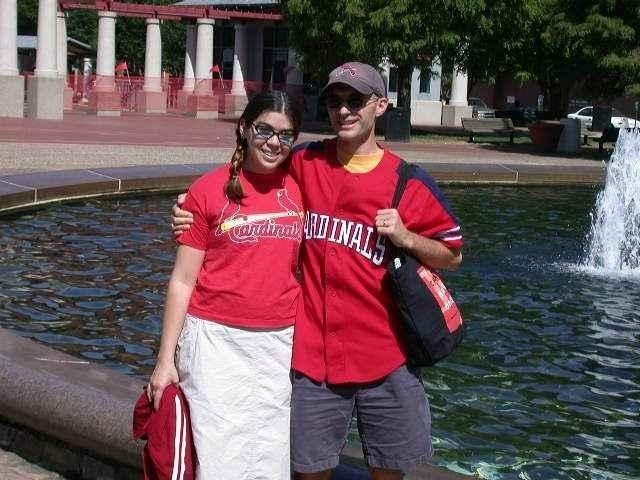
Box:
[592,126,640,153]
[461,117,522,145]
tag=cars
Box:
[496,103,556,126]
[468,96,498,119]
[566,104,640,138]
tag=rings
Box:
[382,219,388,228]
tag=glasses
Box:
[251,121,297,145]
[325,94,379,110]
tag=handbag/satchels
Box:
[388,250,463,367]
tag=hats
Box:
[323,62,385,97]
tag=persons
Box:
[167,54,466,480]
[141,86,309,480]
